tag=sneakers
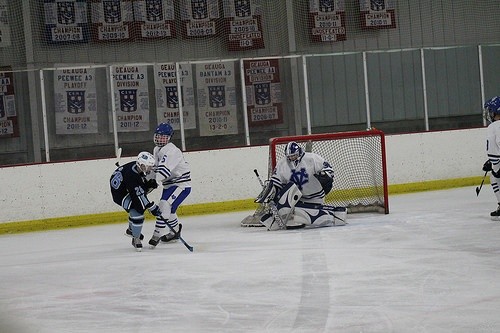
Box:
[148,234,161,248]
[490,202,500,221]
[124,225,144,242]
[131,236,143,252]
[160,223,183,244]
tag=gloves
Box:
[491,168,500,178]
[482,160,492,171]
[146,201,162,216]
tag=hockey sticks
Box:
[116,147,124,167]
[157,212,200,253]
[254,168,295,230]
[476,170,488,196]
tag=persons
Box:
[110,122,192,252]
[254,141,347,230]
[482,95,500,220]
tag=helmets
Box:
[153,122,174,139]
[136,151,155,174]
[284,141,302,158]
[483,95,500,114]
